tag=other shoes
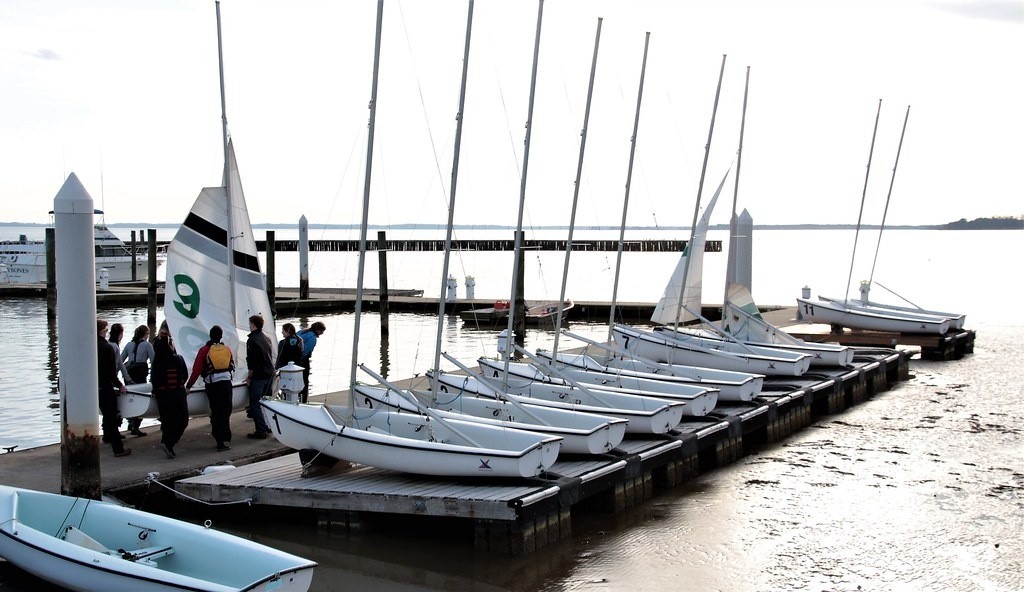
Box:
[247,431,268,440]
[102,434,126,444]
[217,446,232,452]
[114,448,132,457]
[128,425,133,431]
[131,430,147,438]
[159,444,177,459]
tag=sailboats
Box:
[352,0,627,455]
[111,1,278,419]
[481,0,857,417]
[797,98,967,334]
[264,0,563,479]
[426,0,686,435]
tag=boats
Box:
[0,171,167,285]
[504,299,574,324]
[0,484,318,592]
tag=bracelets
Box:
[248,376,251,379]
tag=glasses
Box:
[103,329,108,332]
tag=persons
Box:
[275,323,304,367]
[186,325,235,450]
[121,325,155,436]
[159,319,176,354]
[242,316,275,439]
[97,321,132,457]
[151,332,189,458]
[108,323,134,385]
[297,322,326,403]
